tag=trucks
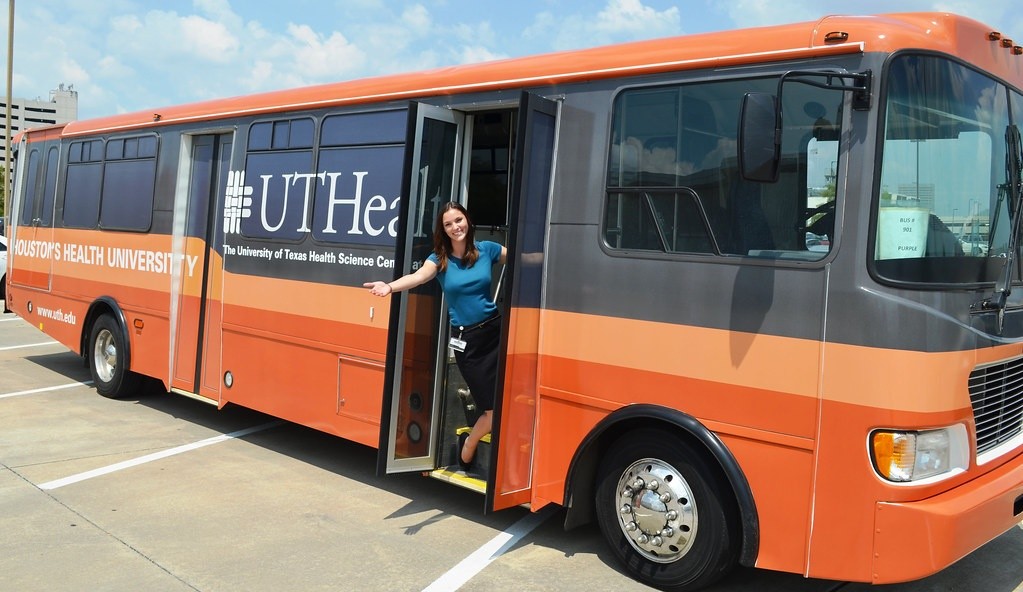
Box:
[874,207,963,260]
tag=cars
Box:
[959,238,988,256]
[0,217,4,236]
[0,234,7,300]
[806,231,830,253]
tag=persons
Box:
[362,201,545,473]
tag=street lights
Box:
[951,208,958,232]
[968,197,981,256]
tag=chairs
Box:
[719,157,773,257]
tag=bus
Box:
[4,12,1023,592]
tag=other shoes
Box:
[457,432,473,471]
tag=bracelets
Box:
[388,285,392,293]
